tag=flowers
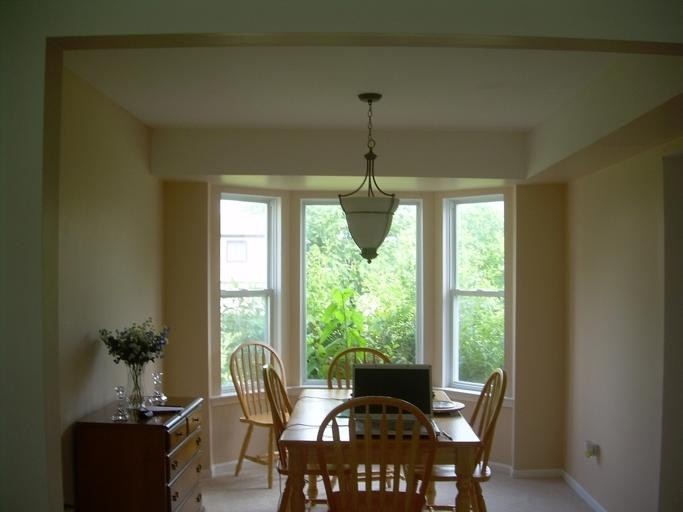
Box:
[98,316,169,406]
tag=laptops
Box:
[352,362,441,436]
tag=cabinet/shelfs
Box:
[71,394,205,512]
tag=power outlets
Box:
[584,441,600,459]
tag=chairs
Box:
[230,340,296,489]
[398,367,507,512]
[315,396,439,512]
[326,348,406,487]
[259,363,352,512]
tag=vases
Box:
[126,364,146,413]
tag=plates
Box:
[433,400,465,414]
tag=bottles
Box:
[147,373,168,406]
[111,386,129,421]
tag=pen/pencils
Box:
[442,431,453,441]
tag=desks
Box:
[279,389,486,512]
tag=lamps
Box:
[338,92,400,264]
